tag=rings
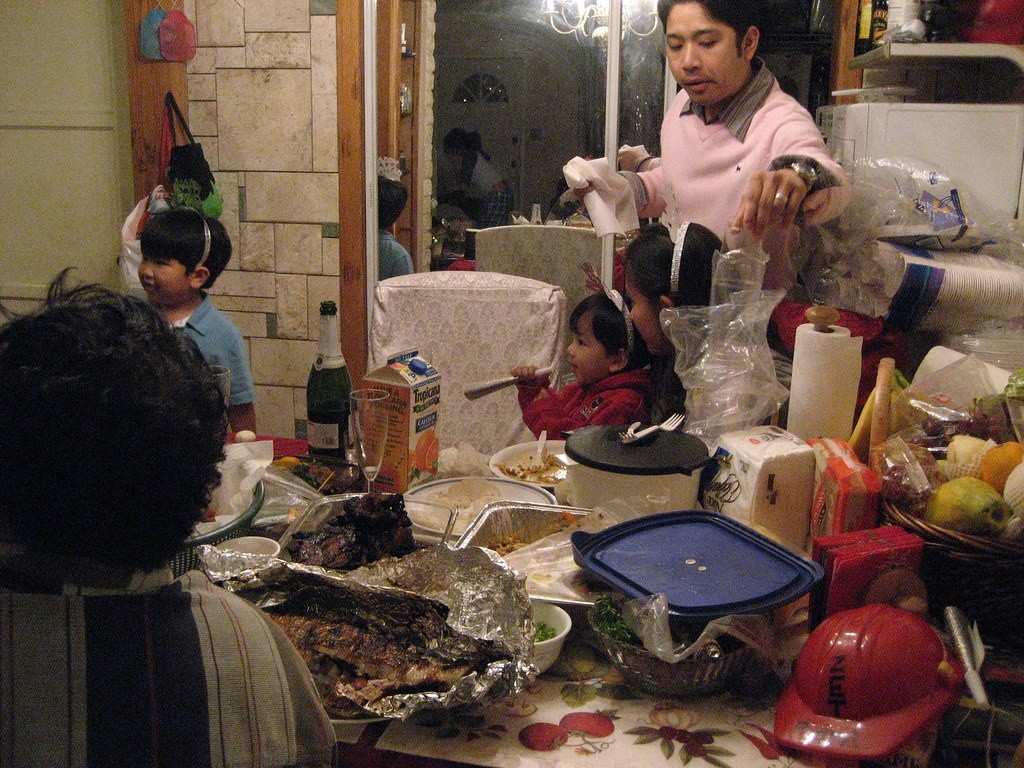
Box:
[775,193,789,203]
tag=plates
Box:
[403,477,558,536]
[489,440,568,487]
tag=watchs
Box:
[784,161,819,197]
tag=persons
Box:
[511,263,652,441]
[0,266,337,768]
[137,209,257,431]
[629,183,780,433]
[546,150,604,223]
[572,0,853,296]
[445,127,513,258]
[377,156,414,279]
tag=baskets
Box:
[878,493,1024,634]
[587,604,750,699]
[168,477,265,580]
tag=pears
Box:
[925,476,1014,539]
[944,433,998,480]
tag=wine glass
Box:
[350,389,390,493]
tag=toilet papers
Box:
[786,323,863,444]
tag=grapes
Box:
[910,411,1006,447]
[878,445,946,516]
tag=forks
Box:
[622,413,685,444]
[530,430,546,472]
[618,422,642,439]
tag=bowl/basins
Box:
[557,424,713,525]
[587,607,771,697]
[215,536,280,557]
[523,599,572,676]
[941,335,1024,374]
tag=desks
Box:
[225,431,308,462]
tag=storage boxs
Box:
[454,500,594,619]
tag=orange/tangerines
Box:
[984,441,1024,493]
[415,430,439,470]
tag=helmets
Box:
[774,603,965,757]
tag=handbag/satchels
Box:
[166,91,223,220]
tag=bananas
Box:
[849,372,913,444]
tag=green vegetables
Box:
[534,621,556,642]
[592,594,640,645]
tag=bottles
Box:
[306,301,353,468]
[855,0,888,58]
[530,203,543,225]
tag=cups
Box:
[209,365,230,413]
[809,226,1024,329]
[464,229,477,260]
